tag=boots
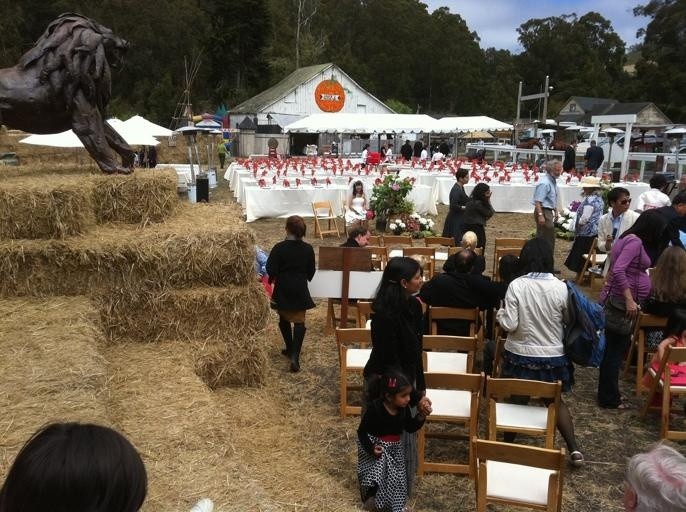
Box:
[278,320,293,358]
[289,326,307,370]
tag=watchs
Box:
[536,212,543,217]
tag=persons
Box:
[338,227,371,248]
[400,140,413,161]
[442,168,495,253]
[564,170,686,410]
[529,159,561,277]
[344,178,369,232]
[385,143,393,162]
[583,140,604,174]
[1,423,148,511]
[380,146,386,158]
[266,216,315,371]
[217,140,232,169]
[414,136,486,164]
[359,371,431,512]
[418,229,585,468]
[363,258,432,417]
[563,140,578,173]
[622,438,686,512]
[252,246,272,301]
[361,144,370,169]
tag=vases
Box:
[375,221,387,232]
[393,230,401,235]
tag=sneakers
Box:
[588,265,601,274]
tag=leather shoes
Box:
[553,269,560,274]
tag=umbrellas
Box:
[18,114,224,163]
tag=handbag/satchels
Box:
[602,293,638,335]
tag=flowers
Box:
[389,219,406,231]
[409,213,434,231]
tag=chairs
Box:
[312,201,340,241]
[575,237,686,442]
[342,200,371,239]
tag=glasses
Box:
[618,199,631,205]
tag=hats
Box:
[576,175,601,188]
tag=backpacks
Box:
[564,277,606,367]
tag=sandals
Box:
[618,392,633,409]
[570,449,585,465]
